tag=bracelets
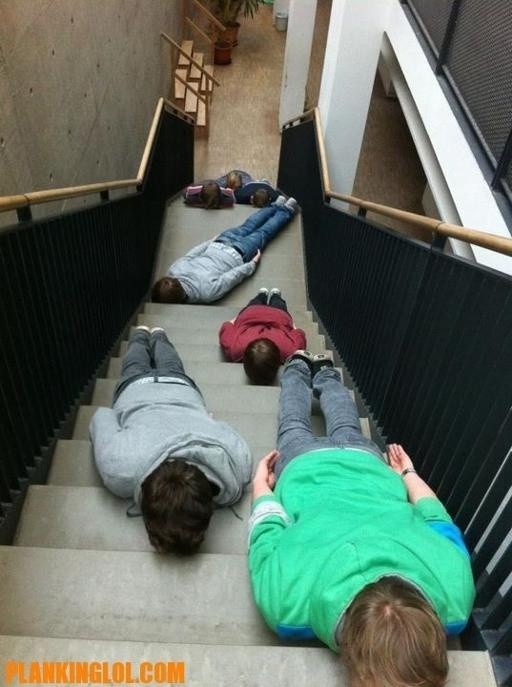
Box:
[400,468,416,477]
[252,260,257,264]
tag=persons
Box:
[183,170,283,209]
[218,288,307,384]
[152,196,301,304]
[249,351,475,687]
[88,325,254,555]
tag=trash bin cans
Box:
[275,13,288,31]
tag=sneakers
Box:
[257,287,269,295]
[135,324,149,334]
[269,287,281,299]
[150,326,167,333]
[284,197,303,213]
[312,353,334,380]
[288,348,313,369]
[275,195,287,206]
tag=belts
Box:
[134,375,192,386]
[212,239,246,263]
